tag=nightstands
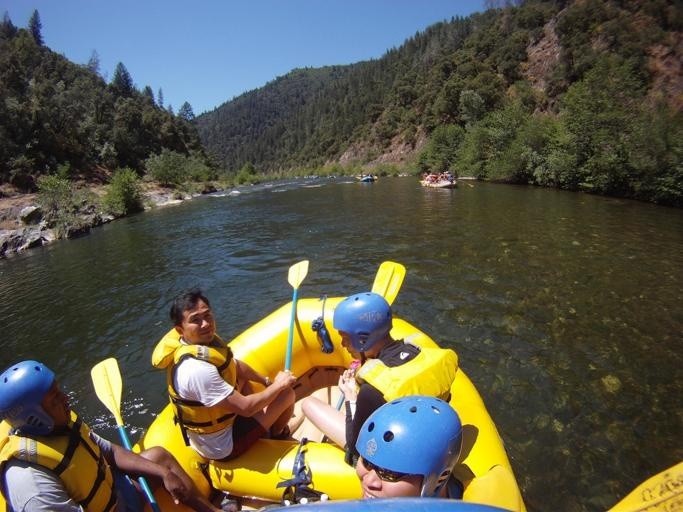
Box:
[456,178,476,189]
[334,261,406,410]
[285,259,308,376]
[90,356,161,510]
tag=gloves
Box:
[415,177,457,189]
[358,176,374,182]
[119,288,529,512]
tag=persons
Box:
[149,286,304,462]
[299,292,458,466]
[422,170,453,185]
[0,360,223,512]
[353,394,462,499]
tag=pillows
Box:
[333,292,393,353]
[1,360,56,436]
[357,395,463,497]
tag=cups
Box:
[362,457,407,482]
